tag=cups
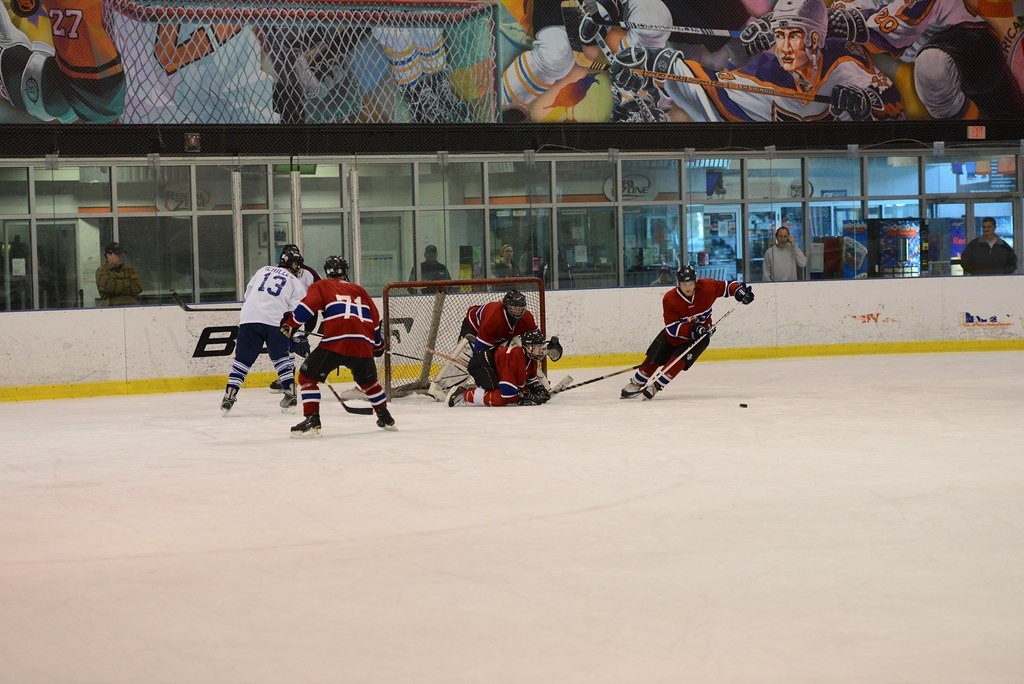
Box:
[697,253,709,265]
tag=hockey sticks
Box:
[621,301,744,398]
[168,288,242,312]
[426,347,573,393]
[548,340,662,396]
[305,352,376,415]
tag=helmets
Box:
[502,289,527,320]
[676,265,696,282]
[521,329,545,361]
[280,245,304,269]
[324,255,349,276]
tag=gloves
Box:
[690,323,716,339]
[518,391,541,405]
[291,334,310,358]
[279,311,300,338]
[528,382,551,399]
[373,338,384,358]
[734,283,755,305]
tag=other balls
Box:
[740,404,747,407]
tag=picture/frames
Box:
[259,222,290,248]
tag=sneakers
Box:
[618,376,645,399]
[290,415,322,438]
[218,395,237,416]
[278,392,298,413]
[444,385,466,408]
[374,413,395,431]
[643,384,660,399]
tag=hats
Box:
[425,245,438,255]
[104,242,126,253]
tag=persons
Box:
[219,252,307,410]
[649,266,674,286]
[960,217,1018,275]
[448,288,550,407]
[407,248,452,295]
[492,243,524,291]
[269,244,321,390]
[620,264,755,399]
[762,226,808,282]
[280,255,395,433]
[96,242,143,307]
[8,234,29,309]
